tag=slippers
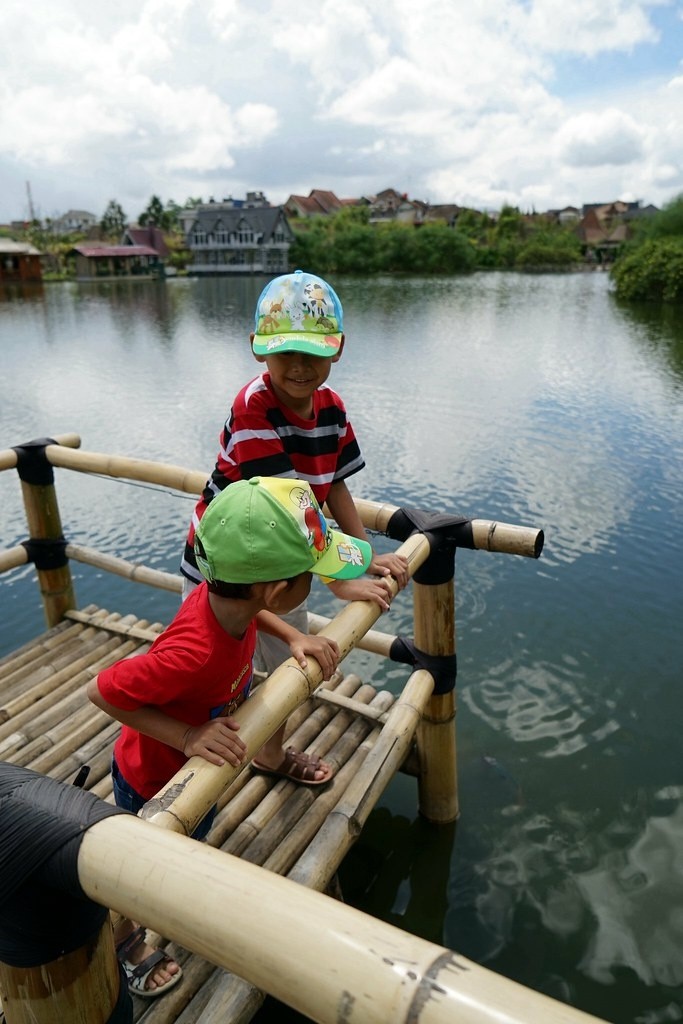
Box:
[249,745,333,785]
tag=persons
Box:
[179,270,408,787]
[85,477,373,997]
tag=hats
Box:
[252,269,343,356]
[194,475,372,585]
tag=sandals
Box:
[118,925,183,999]
